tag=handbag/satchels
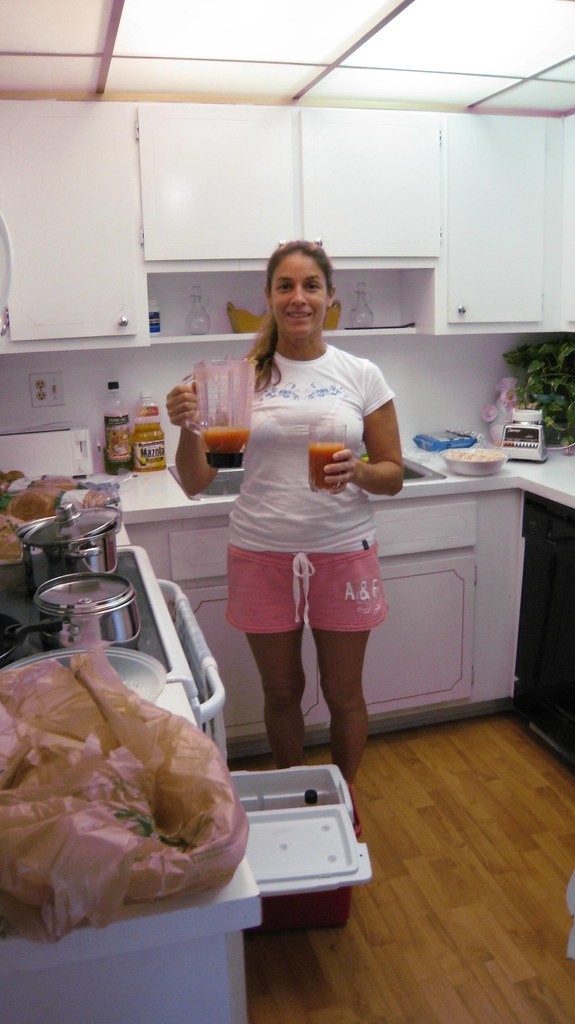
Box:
[0,642,251,947]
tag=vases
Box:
[185,294,209,336]
[348,291,374,328]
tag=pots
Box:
[5,576,141,654]
[16,502,119,597]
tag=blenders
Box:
[501,408,548,462]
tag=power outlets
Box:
[29,371,65,408]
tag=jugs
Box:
[181,359,256,465]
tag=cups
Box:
[309,423,347,493]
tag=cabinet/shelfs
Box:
[122,485,525,763]
[0,680,264,1024]
[0,98,575,355]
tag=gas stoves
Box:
[0,545,198,702]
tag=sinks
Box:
[189,461,424,494]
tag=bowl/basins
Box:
[1,646,166,703]
[440,448,508,476]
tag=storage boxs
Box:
[225,765,372,938]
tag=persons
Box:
[166,241,404,839]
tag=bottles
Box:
[186,285,210,335]
[350,282,375,327]
[131,391,167,471]
[149,299,162,337]
[102,381,132,474]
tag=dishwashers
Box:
[513,492,574,754]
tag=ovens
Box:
[0,422,93,482]
